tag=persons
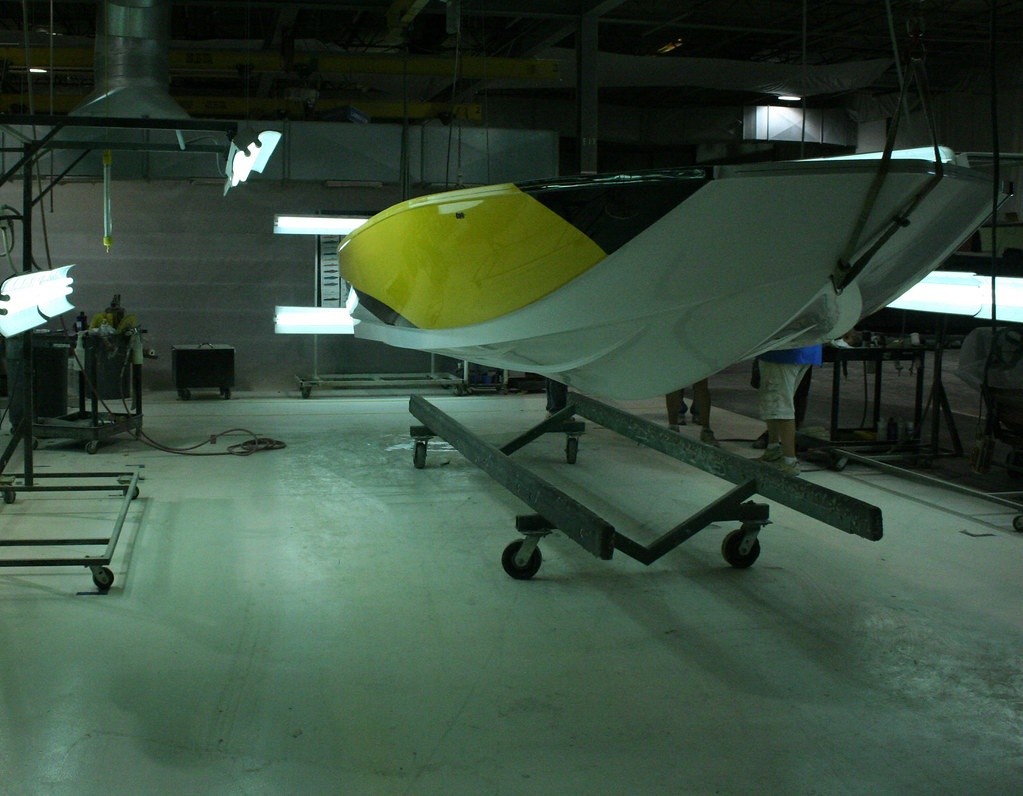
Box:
[758,344,822,475]
[666,378,720,447]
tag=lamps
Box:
[784,1,957,166]
[0,264,78,339]
[221,0,282,196]
[272,212,374,236]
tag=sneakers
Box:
[770,460,799,475]
[749,445,784,463]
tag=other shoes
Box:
[700,431,721,448]
[692,413,703,425]
[676,412,687,425]
[752,430,770,449]
[668,425,679,432]
